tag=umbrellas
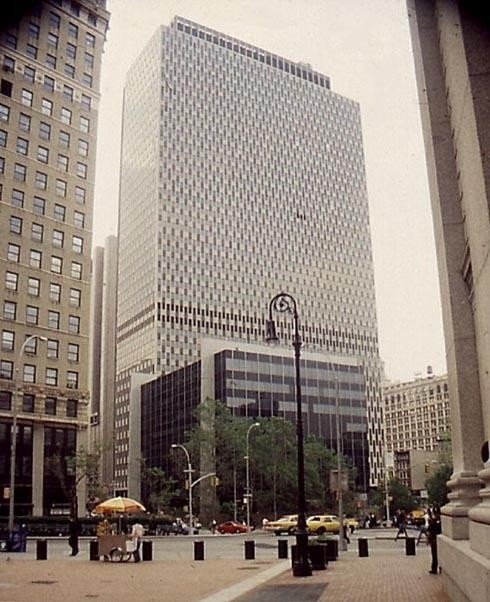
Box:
[91,495,147,535]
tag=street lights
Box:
[246,422,261,533]
[171,444,194,535]
[9,334,48,531]
[266,293,314,577]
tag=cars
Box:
[172,517,199,535]
[264,514,360,535]
[216,521,254,534]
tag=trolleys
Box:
[98,534,138,562]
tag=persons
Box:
[93,513,271,564]
[67,513,83,556]
[331,503,443,574]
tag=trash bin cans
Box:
[11,530,27,552]
